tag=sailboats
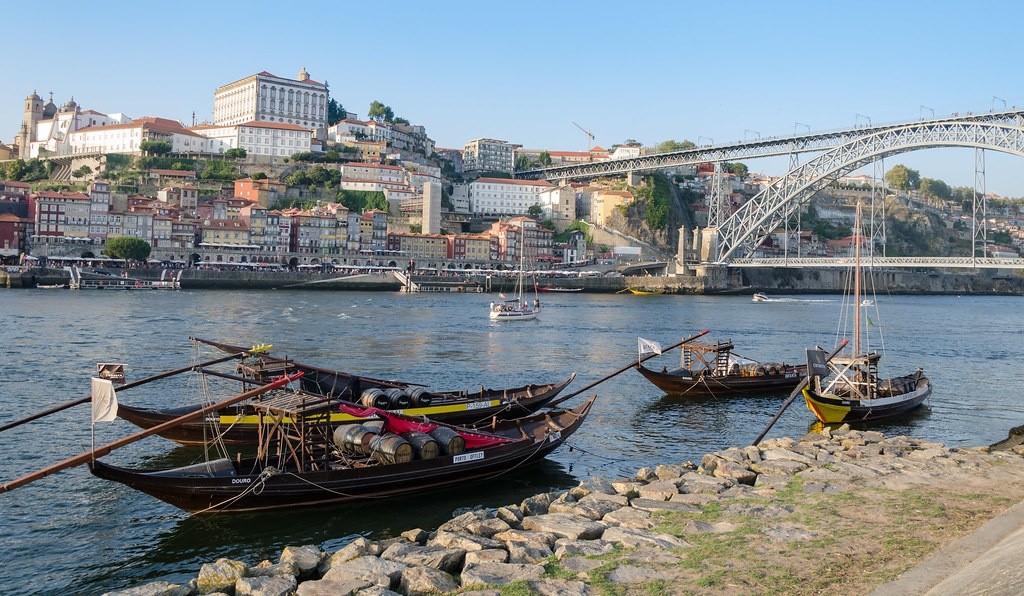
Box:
[490,218,540,321]
[801,200,933,423]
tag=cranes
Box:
[572,121,595,150]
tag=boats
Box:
[752,292,768,302]
[628,285,665,294]
[91,336,577,446]
[88,367,598,515]
[37,283,65,288]
[541,286,584,292]
[634,338,836,397]
[863,300,873,305]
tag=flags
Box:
[499,293,506,298]
[97,363,126,384]
[638,337,661,355]
[92,378,118,424]
[806,350,829,376]
